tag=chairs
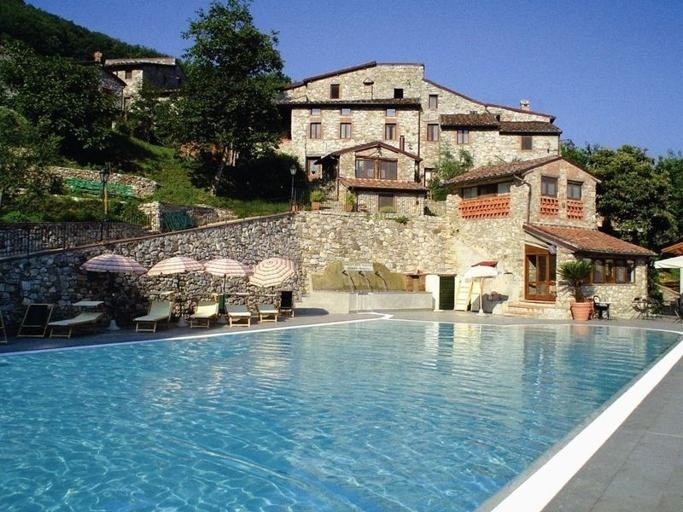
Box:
[45,292,296,339]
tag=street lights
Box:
[98,166,110,222]
[289,162,298,211]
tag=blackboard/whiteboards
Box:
[16,304,54,337]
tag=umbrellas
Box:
[143,256,203,315]
[77,251,147,277]
[247,255,298,293]
[195,257,252,295]
[461,264,497,309]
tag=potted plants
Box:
[555,258,594,321]
[309,190,322,210]
[342,191,355,213]
